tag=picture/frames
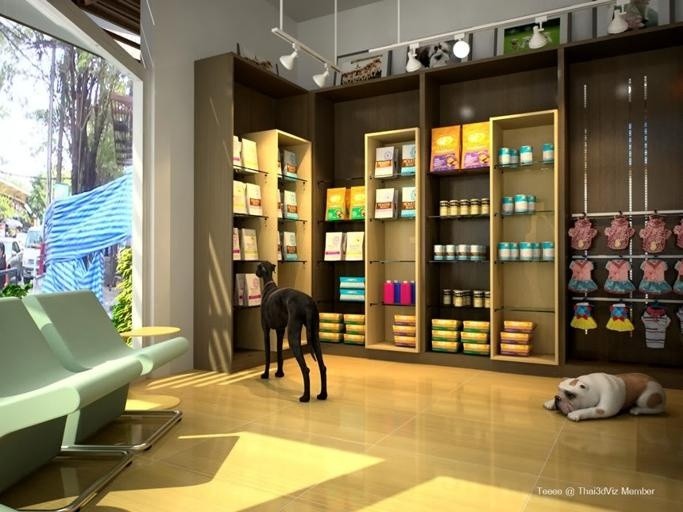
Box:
[331,46,391,87]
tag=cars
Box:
[12,216,31,232]
[1,238,20,285]
[0,217,22,232]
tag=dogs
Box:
[255,259,328,403]
[542,371,666,422]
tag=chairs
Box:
[21,290,188,452]
[0,295,134,511]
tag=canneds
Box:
[498,142,554,167]
[442,287,490,309]
[439,197,490,217]
[497,241,555,262]
[501,193,537,216]
[433,243,487,262]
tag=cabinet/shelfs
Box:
[427,155,490,316]
[492,156,556,319]
[313,176,367,309]
[274,164,307,267]
[567,207,682,309]
[227,158,269,309]
[370,172,416,309]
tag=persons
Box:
[0,241,7,291]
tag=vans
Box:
[21,227,41,281]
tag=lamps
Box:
[367,1,635,74]
[268,1,342,90]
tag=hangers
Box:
[571,251,671,263]
[571,291,679,309]
[570,209,682,222]
